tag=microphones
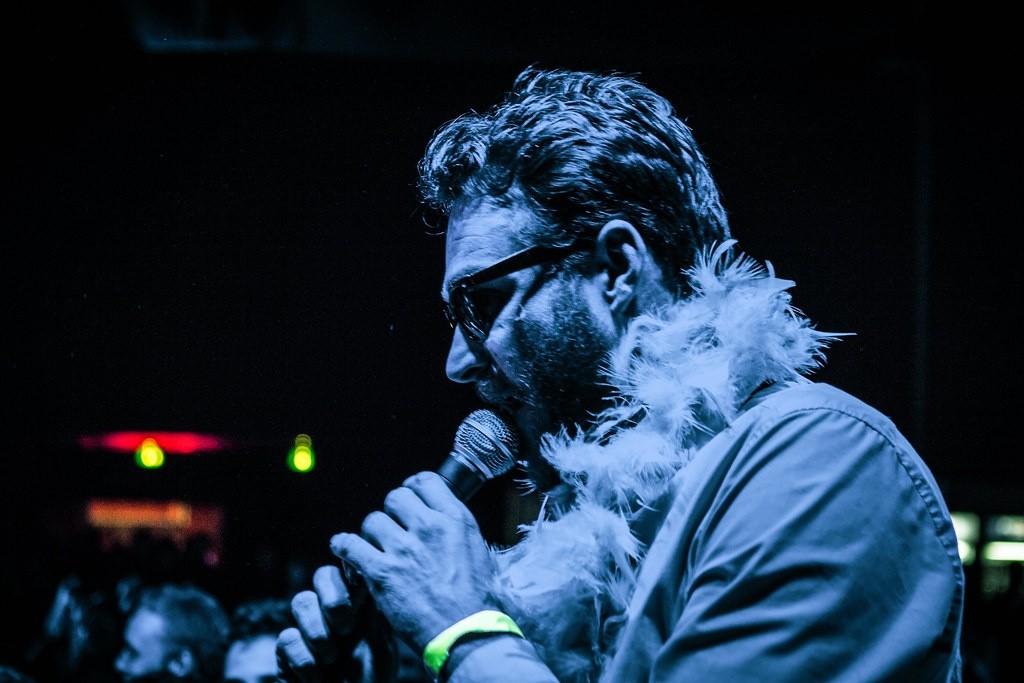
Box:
[283,405,522,683]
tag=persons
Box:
[0,520,372,683]
[276,64,965,683]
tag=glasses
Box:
[441,236,598,344]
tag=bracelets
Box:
[424,610,525,683]
